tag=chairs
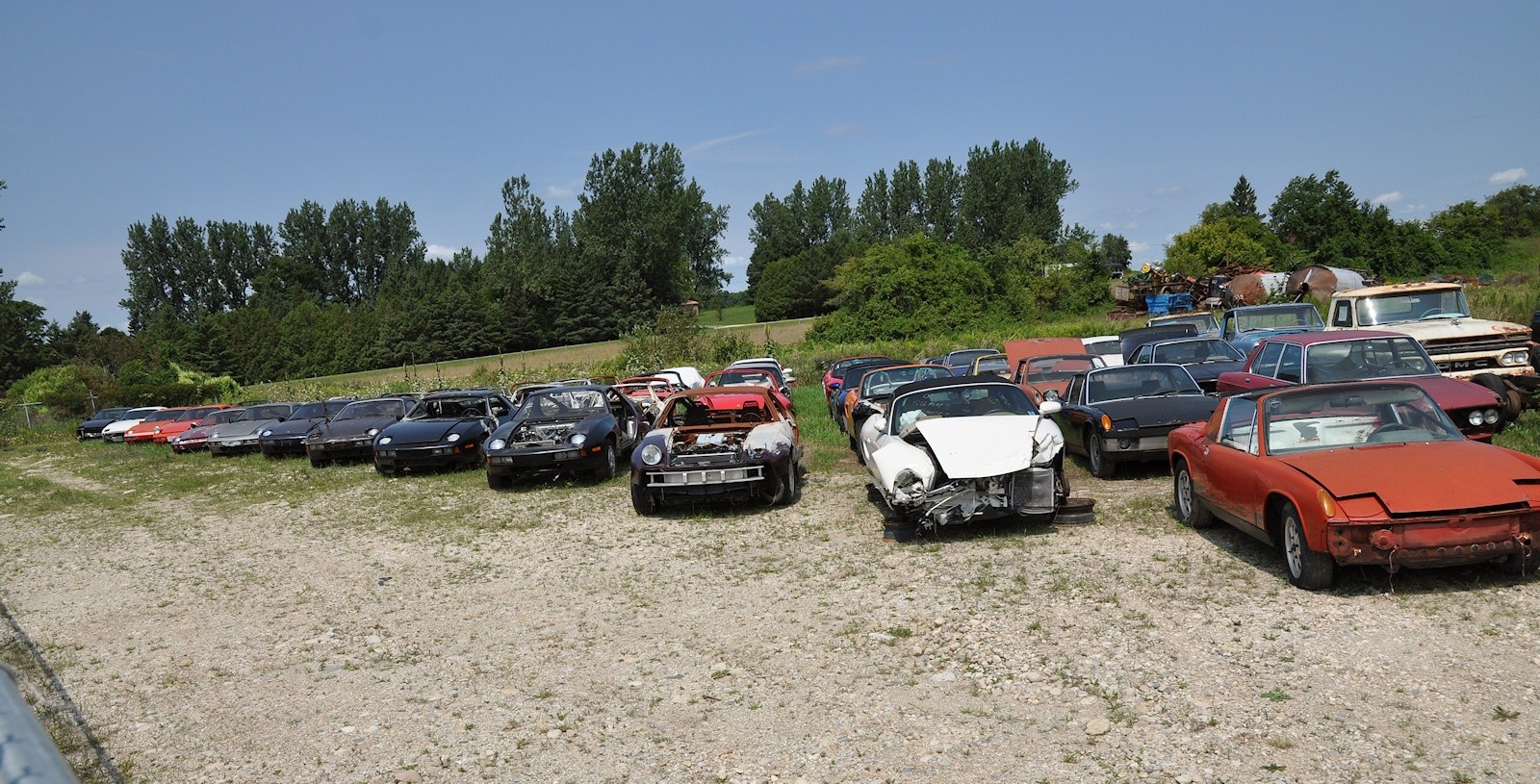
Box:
[741,399,760,422]
[1089,380,1107,402]
[975,396,1003,414]
[686,401,710,423]
[905,395,931,411]
[1142,379,1160,394]
[1194,346,1211,359]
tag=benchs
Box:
[1232,415,1378,451]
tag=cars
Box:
[1118,311,1252,392]
[822,355,965,463]
[694,356,797,415]
[1168,379,1540,593]
[305,393,429,468]
[482,385,648,489]
[125,406,248,454]
[76,406,165,443]
[507,367,710,427]
[1045,362,1222,477]
[373,389,519,477]
[1216,303,1324,360]
[208,399,303,456]
[858,378,1072,536]
[922,348,1012,383]
[1216,328,1505,446]
[258,396,360,459]
[629,385,805,515]
[1000,338,1108,409]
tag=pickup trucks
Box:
[1324,281,1540,434]
